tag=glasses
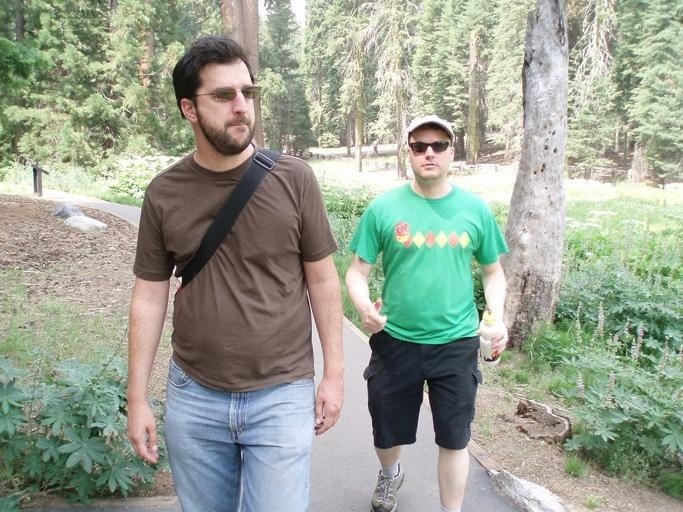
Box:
[190,86,261,102]
[409,141,450,154]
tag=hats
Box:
[405,114,455,139]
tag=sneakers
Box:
[372,464,404,511]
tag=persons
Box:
[345,116,509,512]
[125,35,342,512]
